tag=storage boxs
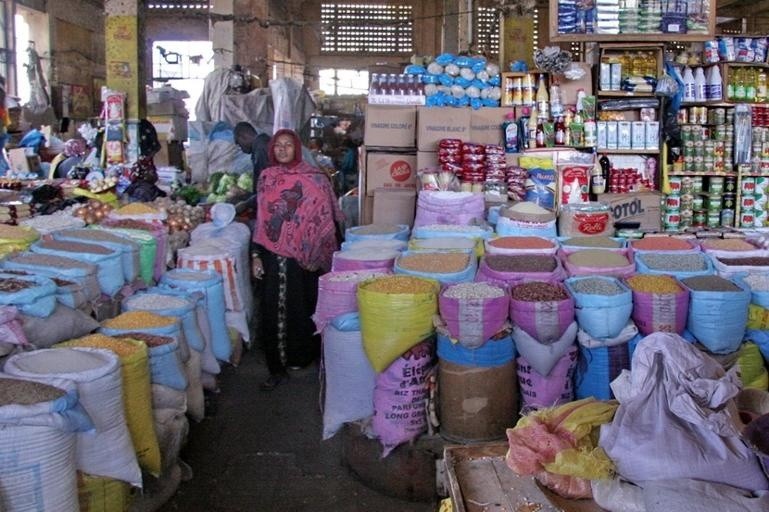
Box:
[595,189,663,234]
[144,102,188,167]
[365,103,662,232]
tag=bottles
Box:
[674,65,722,102]
[589,150,611,196]
[500,73,597,153]
[621,50,656,75]
[310,113,317,128]
[727,65,769,103]
[367,73,424,96]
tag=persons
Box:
[225,120,271,216]
[246,126,348,395]
[322,114,364,195]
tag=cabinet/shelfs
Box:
[593,35,769,231]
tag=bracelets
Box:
[250,252,262,259]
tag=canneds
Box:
[503,74,536,105]
[665,106,769,228]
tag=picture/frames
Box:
[549,0,716,42]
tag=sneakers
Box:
[286,359,311,371]
[260,373,289,391]
